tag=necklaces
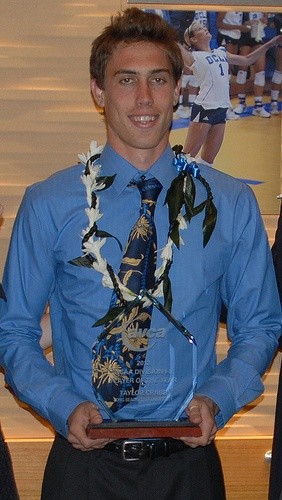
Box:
[82,145,198,331]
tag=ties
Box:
[92,176,164,413]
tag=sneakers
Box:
[226,110,240,120]
[175,108,189,118]
[233,104,247,113]
[252,107,271,118]
[270,105,279,115]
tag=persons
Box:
[148,8,281,118]
[0,9,280,500]
[172,23,281,164]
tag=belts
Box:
[102,440,189,461]
